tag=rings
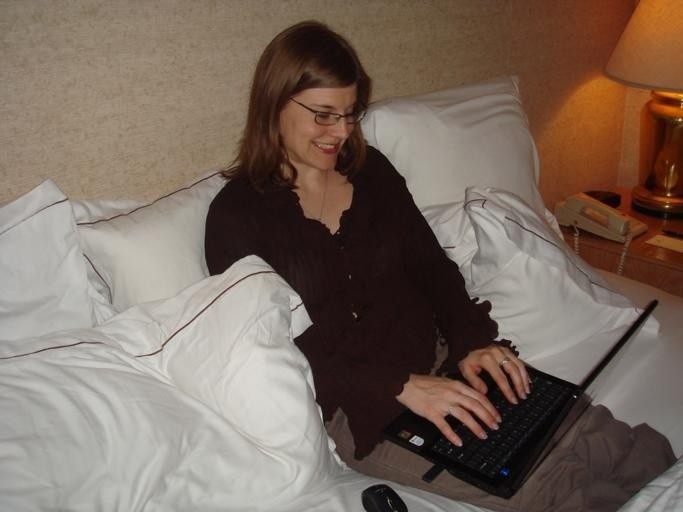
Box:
[500,357,512,365]
[447,403,457,413]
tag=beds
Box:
[0,172,682,511]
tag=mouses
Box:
[361,484,408,512]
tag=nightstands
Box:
[557,186,683,297]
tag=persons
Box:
[205,20,677,512]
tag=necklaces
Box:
[295,170,330,221]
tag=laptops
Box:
[381,298,659,501]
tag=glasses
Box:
[291,98,367,126]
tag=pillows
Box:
[359,74,564,238]
[420,187,659,360]
[2,179,118,342]
[0,255,346,510]
[73,164,237,312]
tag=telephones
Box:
[555,192,647,243]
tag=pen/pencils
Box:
[662,229,683,238]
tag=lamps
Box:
[605,1,683,219]
